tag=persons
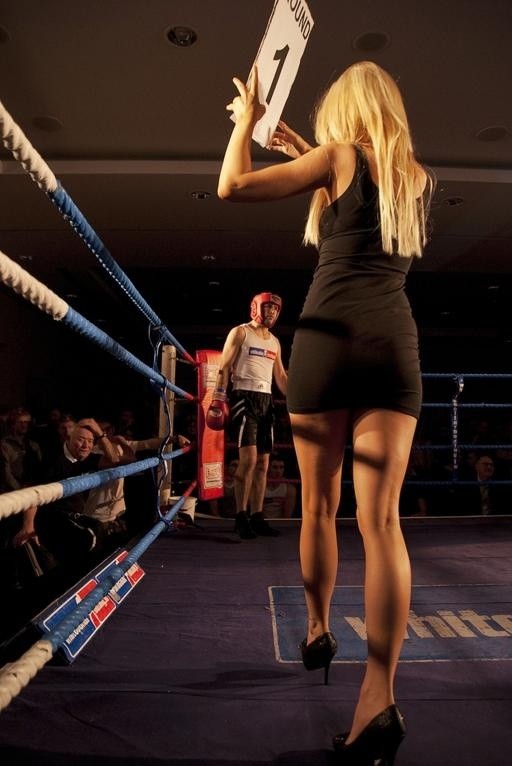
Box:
[216,59,436,765]
[1,408,512,548]
[206,292,289,541]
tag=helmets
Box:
[249,292,282,328]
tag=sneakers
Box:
[233,511,257,540]
[249,512,280,538]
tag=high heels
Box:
[302,631,336,686]
[331,704,406,765]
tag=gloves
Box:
[206,392,231,431]
